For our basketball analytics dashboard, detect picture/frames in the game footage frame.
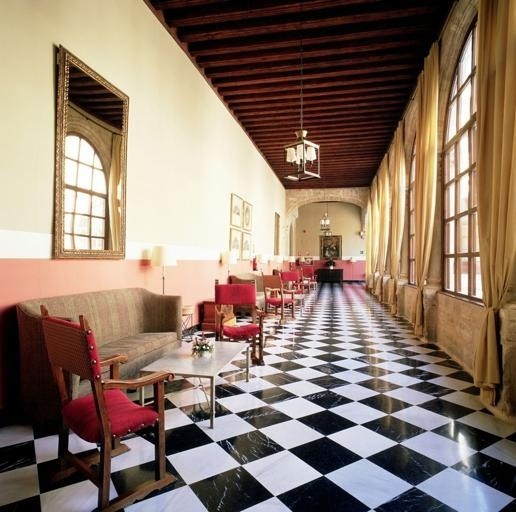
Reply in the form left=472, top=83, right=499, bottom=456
left=319, top=234, right=343, bottom=260
left=227, top=192, right=256, bottom=263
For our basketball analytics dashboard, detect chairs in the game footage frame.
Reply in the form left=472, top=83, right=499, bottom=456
left=38, top=303, right=178, bottom=512
left=213, top=279, right=265, bottom=367
left=262, top=262, right=319, bottom=326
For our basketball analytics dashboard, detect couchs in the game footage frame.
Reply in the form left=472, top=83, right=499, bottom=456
left=15, top=286, right=185, bottom=437
left=229, top=271, right=271, bottom=317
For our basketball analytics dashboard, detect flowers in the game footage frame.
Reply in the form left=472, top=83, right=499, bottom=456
left=190, top=332, right=214, bottom=362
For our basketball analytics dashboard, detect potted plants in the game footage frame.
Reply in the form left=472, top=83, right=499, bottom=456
left=325, top=259, right=336, bottom=271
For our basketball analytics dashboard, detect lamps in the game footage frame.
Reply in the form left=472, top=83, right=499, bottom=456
left=358, top=229, right=366, bottom=240
left=318, top=201, right=333, bottom=240
left=142, top=242, right=179, bottom=297
left=282, top=1, right=324, bottom=185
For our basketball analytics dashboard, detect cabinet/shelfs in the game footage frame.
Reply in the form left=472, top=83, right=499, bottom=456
left=319, top=268, right=344, bottom=289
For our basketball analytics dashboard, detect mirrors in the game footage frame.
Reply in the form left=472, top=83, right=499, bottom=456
left=53, top=44, right=129, bottom=260
